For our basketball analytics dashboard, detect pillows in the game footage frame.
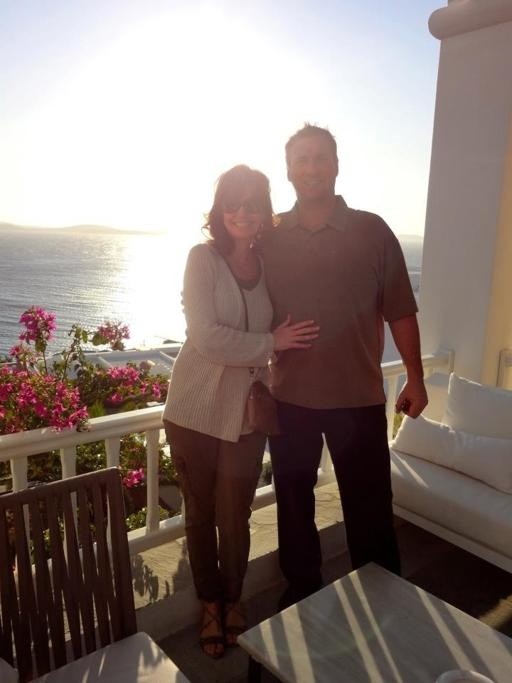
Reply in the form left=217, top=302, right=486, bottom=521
left=388, top=373, right=511, bottom=497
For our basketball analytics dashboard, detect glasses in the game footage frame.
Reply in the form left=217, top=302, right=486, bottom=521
left=216, top=198, right=270, bottom=214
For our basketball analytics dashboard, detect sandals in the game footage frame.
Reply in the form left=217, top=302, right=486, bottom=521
left=225, top=602, right=245, bottom=648
left=200, top=601, right=225, bottom=657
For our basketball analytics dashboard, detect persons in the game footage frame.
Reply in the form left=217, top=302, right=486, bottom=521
left=161, top=164, right=321, bottom=661
left=180, top=121, right=427, bottom=613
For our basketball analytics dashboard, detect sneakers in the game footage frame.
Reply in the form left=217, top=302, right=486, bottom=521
left=277, top=578, right=315, bottom=613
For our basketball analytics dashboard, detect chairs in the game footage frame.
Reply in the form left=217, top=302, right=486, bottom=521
left=387, top=371, right=511, bottom=575
left=0, top=465, right=193, bottom=682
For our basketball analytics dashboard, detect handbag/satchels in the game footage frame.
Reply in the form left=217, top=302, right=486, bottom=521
left=246, top=380, right=281, bottom=437
